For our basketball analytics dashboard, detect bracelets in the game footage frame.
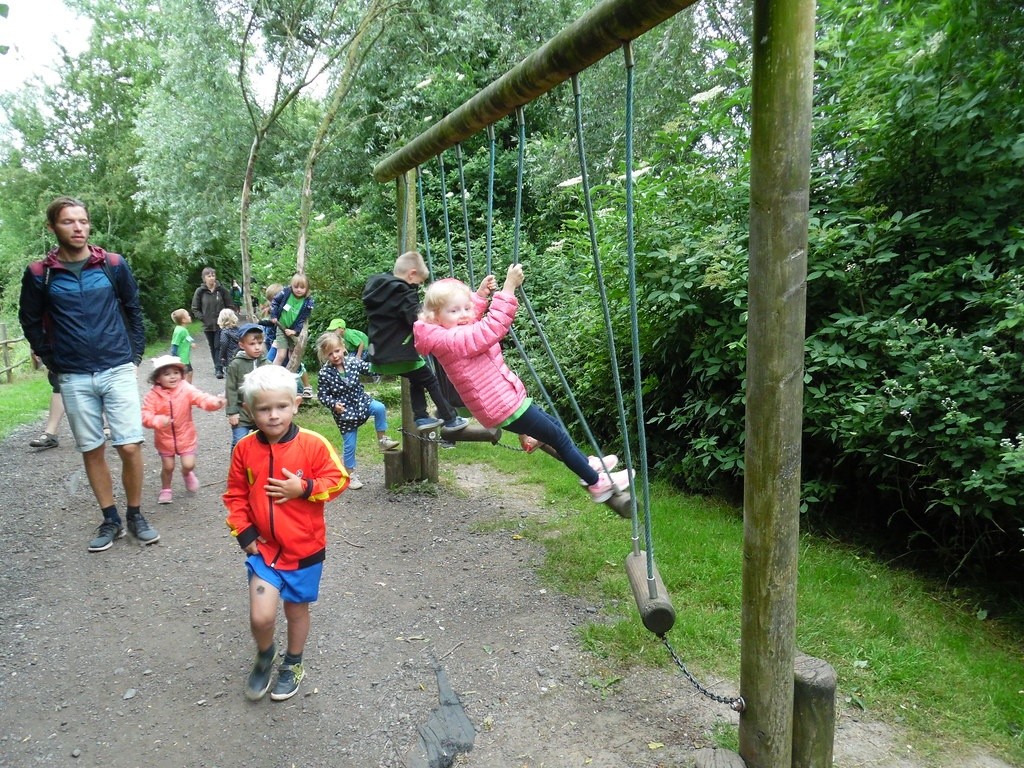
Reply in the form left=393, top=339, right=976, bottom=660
left=301, top=478, right=314, bottom=500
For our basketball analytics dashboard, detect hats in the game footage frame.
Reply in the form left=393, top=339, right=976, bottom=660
left=326, top=318, right=346, bottom=331
left=239, top=323, right=263, bottom=340
left=146, top=355, right=185, bottom=384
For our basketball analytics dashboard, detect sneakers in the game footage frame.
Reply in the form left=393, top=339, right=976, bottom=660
left=378, top=435, right=400, bottom=451
left=416, top=415, right=444, bottom=431
left=442, top=415, right=469, bottom=432
left=184, top=470, right=199, bottom=491
left=88, top=518, right=126, bottom=551
left=271, top=663, right=305, bottom=700
left=348, top=472, right=363, bottom=490
left=159, top=488, right=174, bottom=504
left=125, top=512, right=160, bottom=544
left=579, top=454, right=618, bottom=486
left=588, top=468, right=635, bottom=503
left=245, top=652, right=278, bottom=702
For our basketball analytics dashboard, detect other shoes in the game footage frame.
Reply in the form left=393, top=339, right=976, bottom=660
left=216, top=370, right=224, bottom=378
left=522, top=437, right=545, bottom=453
left=373, top=375, right=380, bottom=383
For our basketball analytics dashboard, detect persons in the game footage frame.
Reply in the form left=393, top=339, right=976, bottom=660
left=17, top=195, right=161, bottom=552
left=230, top=278, right=242, bottom=316
left=142, top=355, right=228, bottom=505
left=29, top=371, right=65, bottom=450
left=269, top=272, right=314, bottom=365
left=258, top=282, right=313, bottom=399
left=250, top=277, right=260, bottom=313
left=192, top=267, right=234, bottom=379
left=413, top=263, right=636, bottom=503
left=363, top=250, right=470, bottom=435
left=326, top=318, right=381, bottom=384
left=224, top=365, right=352, bottom=702
left=217, top=309, right=273, bottom=461
left=170, top=308, right=196, bottom=384
left=316, top=331, right=401, bottom=490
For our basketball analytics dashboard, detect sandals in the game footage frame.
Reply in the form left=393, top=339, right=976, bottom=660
left=302, top=385, right=313, bottom=396
left=31, top=432, right=59, bottom=447
left=103, top=425, right=112, bottom=441
